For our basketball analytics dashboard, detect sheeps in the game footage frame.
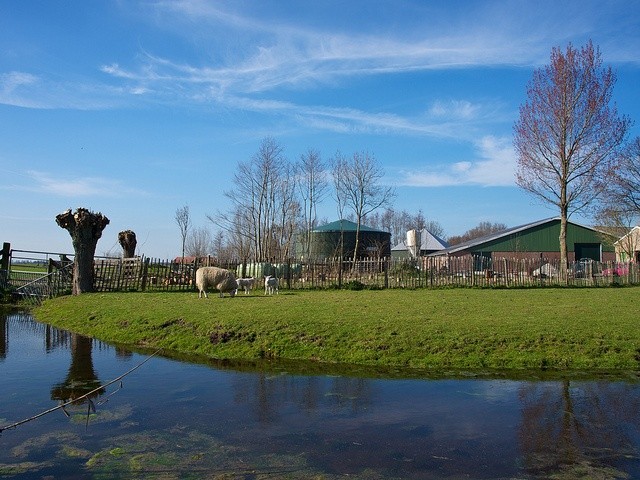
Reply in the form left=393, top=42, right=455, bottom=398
left=263, top=275, right=279, bottom=295
left=196, top=267, right=238, bottom=299
left=235, top=275, right=256, bottom=295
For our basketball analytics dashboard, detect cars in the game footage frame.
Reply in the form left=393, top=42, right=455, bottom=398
left=602, top=263, right=630, bottom=277
